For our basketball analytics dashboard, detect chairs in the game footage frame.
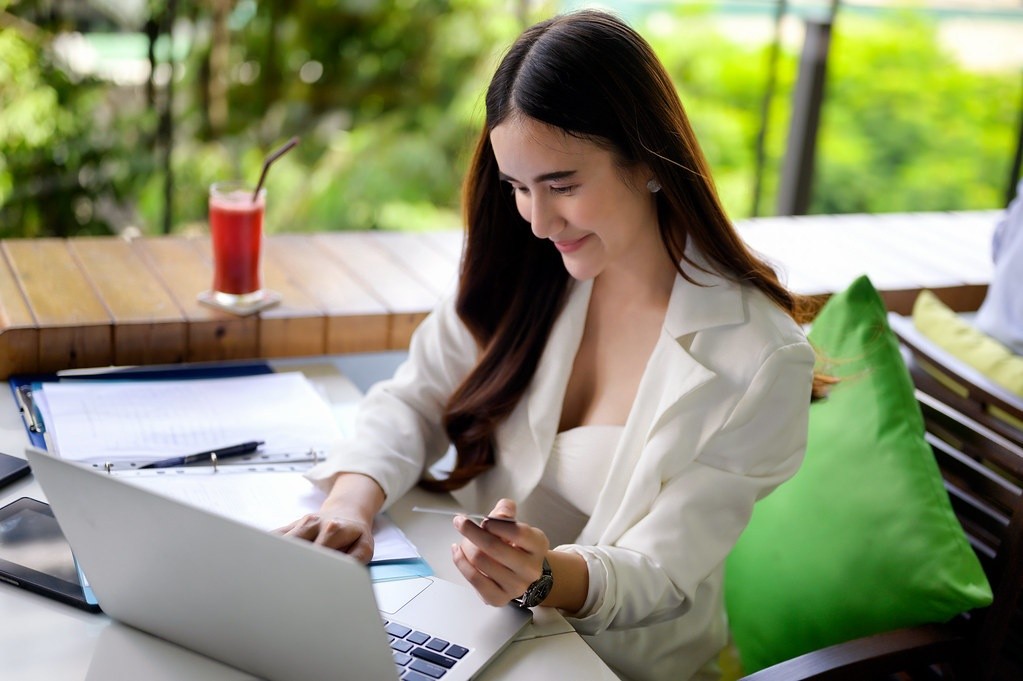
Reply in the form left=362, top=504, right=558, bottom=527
left=709, top=311, right=1023, bottom=681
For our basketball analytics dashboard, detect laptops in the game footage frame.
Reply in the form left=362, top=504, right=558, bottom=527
left=24, top=444, right=534, bottom=680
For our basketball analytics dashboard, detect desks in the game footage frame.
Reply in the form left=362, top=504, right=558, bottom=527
left=0, top=362, right=619, bottom=681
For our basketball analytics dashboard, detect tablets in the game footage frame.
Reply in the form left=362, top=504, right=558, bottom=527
left=0, top=497, right=109, bottom=615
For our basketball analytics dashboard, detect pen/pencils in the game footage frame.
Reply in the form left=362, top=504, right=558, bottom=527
left=137, top=437, right=266, bottom=470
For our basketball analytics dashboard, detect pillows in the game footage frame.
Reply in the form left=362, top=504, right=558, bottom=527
left=913, top=287, right=1023, bottom=478
left=698, top=276, right=993, bottom=672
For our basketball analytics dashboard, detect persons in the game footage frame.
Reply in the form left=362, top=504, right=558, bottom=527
left=268, top=9, right=835, bottom=681
left=975, top=178, right=1023, bottom=355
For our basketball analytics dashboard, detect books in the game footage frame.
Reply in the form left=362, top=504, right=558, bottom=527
left=37, top=371, right=432, bottom=606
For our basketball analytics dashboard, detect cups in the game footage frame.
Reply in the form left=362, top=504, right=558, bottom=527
left=207, top=181, right=266, bottom=305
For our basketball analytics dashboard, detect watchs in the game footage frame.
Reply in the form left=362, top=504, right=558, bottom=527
left=513, top=556, right=554, bottom=608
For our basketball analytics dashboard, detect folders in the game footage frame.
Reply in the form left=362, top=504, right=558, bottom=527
left=7, top=361, right=433, bottom=605
